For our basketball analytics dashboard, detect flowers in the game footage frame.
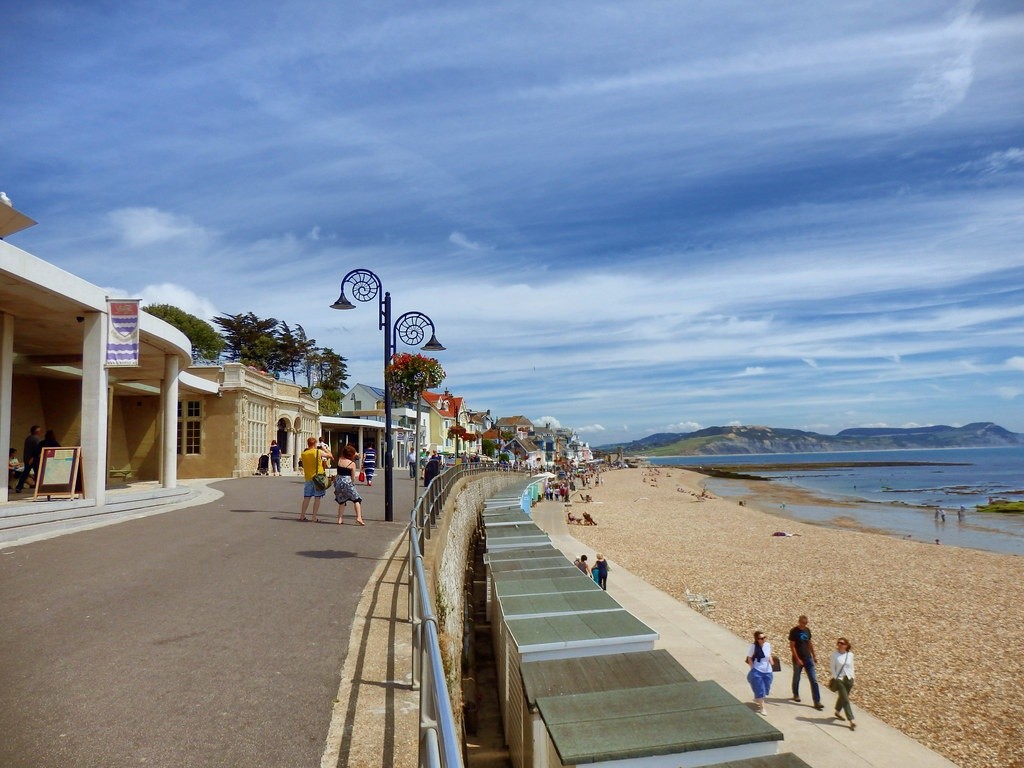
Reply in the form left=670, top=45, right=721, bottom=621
left=384, top=351, right=447, bottom=408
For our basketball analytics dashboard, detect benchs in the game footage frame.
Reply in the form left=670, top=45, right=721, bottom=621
left=109, top=468, right=138, bottom=483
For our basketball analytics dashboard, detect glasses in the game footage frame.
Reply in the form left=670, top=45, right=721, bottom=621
left=758, top=636, right=766, bottom=640
left=837, top=641, right=847, bottom=645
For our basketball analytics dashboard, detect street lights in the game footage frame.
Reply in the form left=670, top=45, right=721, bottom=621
left=438, top=397, right=475, bottom=458
left=329, top=266, right=448, bottom=522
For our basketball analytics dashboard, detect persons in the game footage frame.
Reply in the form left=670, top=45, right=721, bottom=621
left=545, top=463, right=602, bottom=501
left=299, top=437, right=332, bottom=523
left=362, top=443, right=377, bottom=486
left=787, top=615, right=824, bottom=708
left=745, top=631, right=774, bottom=716
left=591, top=553, right=608, bottom=590
left=8, top=424, right=62, bottom=493
left=461, top=450, right=480, bottom=469
left=830, top=637, right=856, bottom=730
left=419, top=448, right=442, bottom=480
left=773, top=531, right=802, bottom=536
left=934, top=505, right=965, bottom=522
left=316, top=437, right=328, bottom=461
left=495, top=459, right=508, bottom=468
left=333, top=446, right=366, bottom=526
left=406, top=447, right=416, bottom=480
left=267, top=439, right=282, bottom=476
left=576, top=555, right=591, bottom=575
left=568, top=512, right=597, bottom=525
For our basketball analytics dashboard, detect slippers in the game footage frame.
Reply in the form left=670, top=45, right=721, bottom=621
left=299, top=517, right=310, bottom=522
left=311, top=518, right=321, bottom=522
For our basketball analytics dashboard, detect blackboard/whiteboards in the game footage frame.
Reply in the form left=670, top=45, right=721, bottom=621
left=34, top=447, right=85, bottom=496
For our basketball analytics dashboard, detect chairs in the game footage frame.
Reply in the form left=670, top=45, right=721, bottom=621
left=584, top=515, right=593, bottom=525
left=580, top=493, right=592, bottom=503
left=566, top=513, right=583, bottom=525
left=683, top=586, right=718, bottom=616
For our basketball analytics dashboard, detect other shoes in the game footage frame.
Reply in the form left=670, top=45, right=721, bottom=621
left=794, top=694, right=801, bottom=701
left=814, top=702, right=824, bottom=708
left=367, top=482, right=370, bottom=486
left=355, top=518, right=365, bottom=526
left=761, top=708, right=767, bottom=716
left=851, top=723, right=856, bottom=730
left=338, top=520, right=343, bottom=524
left=835, top=712, right=845, bottom=720
left=755, top=705, right=760, bottom=712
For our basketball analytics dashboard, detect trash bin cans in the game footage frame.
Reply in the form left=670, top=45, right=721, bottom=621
left=424, top=461, right=441, bottom=487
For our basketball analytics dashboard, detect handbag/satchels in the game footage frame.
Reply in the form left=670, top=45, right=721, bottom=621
left=312, top=474, right=332, bottom=491
left=829, top=678, right=838, bottom=692
left=359, top=468, right=365, bottom=481
left=606, top=564, right=611, bottom=572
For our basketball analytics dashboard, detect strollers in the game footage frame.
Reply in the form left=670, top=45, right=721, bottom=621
left=254, top=454, right=269, bottom=476
